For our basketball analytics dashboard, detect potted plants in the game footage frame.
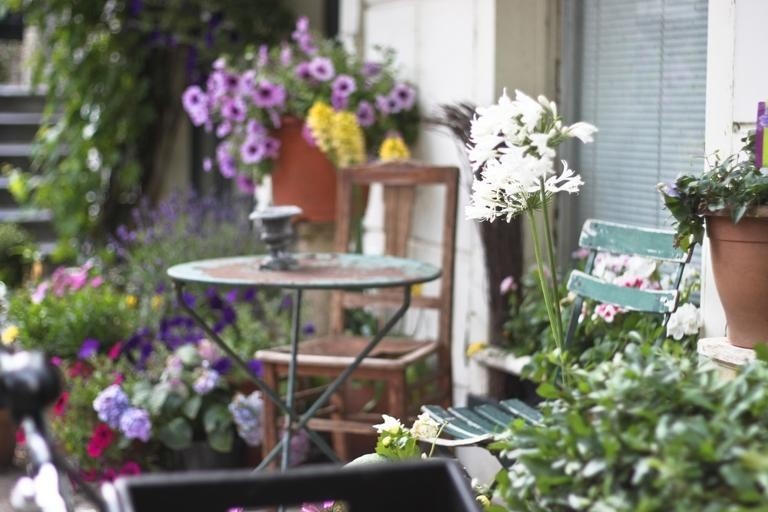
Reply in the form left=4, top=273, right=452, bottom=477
left=656, top=146, right=768, bottom=347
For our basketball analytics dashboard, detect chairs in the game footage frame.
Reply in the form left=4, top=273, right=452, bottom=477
left=420, top=219, right=696, bottom=450
